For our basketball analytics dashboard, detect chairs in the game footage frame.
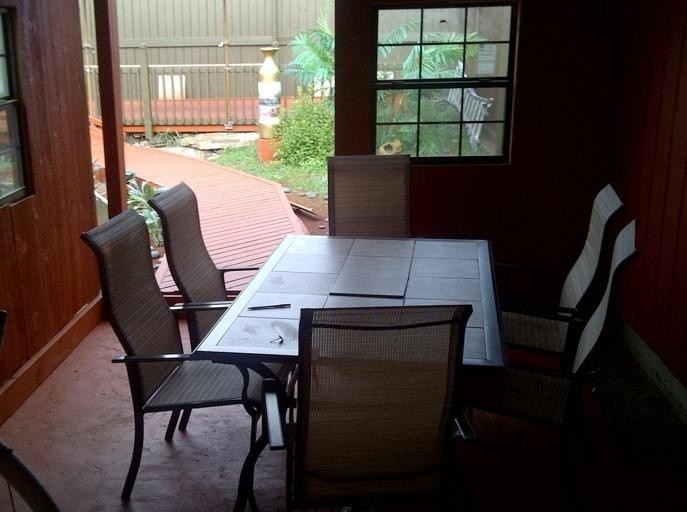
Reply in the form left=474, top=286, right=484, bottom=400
left=260, top=306, right=481, bottom=512
left=498, top=180, right=624, bottom=372
left=470, top=217, right=641, bottom=480
left=79, top=210, right=290, bottom=511
left=151, top=182, right=290, bottom=430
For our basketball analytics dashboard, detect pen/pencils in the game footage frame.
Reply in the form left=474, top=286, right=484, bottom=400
left=248, top=304, right=291, bottom=309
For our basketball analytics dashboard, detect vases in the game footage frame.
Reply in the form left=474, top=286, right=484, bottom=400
left=257, top=47, right=281, bottom=138
